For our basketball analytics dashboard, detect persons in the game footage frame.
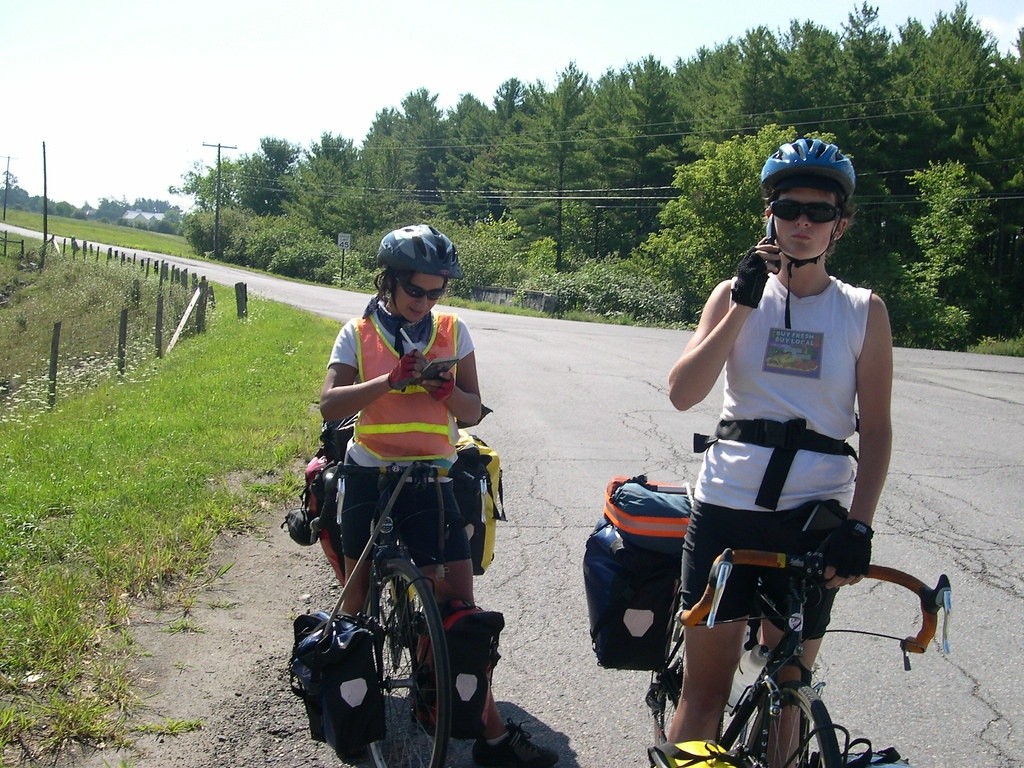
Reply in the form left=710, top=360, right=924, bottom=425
left=668, top=139, right=893, bottom=768
left=319, top=223, right=560, bottom=768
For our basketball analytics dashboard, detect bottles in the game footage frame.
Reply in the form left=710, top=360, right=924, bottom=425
left=724, top=644, right=770, bottom=715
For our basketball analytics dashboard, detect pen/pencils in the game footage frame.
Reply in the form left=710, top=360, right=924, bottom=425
left=400, top=327, right=430, bottom=364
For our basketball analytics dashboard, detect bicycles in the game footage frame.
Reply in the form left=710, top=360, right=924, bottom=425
left=645, top=548, right=954, bottom=767
left=322, top=460, right=487, bottom=767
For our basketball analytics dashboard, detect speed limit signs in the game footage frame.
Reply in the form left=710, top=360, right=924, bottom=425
left=338, top=234, right=351, bottom=249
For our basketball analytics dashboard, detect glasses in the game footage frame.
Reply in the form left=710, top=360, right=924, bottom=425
left=770, top=200, right=843, bottom=224
left=395, top=275, right=445, bottom=302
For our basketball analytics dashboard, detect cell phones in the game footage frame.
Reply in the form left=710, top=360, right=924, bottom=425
left=766, top=213, right=781, bottom=275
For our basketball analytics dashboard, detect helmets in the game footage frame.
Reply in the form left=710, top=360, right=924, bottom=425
left=378, top=224, right=463, bottom=280
left=760, top=139, right=856, bottom=205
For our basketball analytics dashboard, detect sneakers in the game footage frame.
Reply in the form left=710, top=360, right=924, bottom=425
left=473, top=723, right=559, bottom=768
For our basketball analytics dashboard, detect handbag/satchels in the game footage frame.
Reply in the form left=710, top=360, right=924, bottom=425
left=288, top=608, right=388, bottom=755
left=414, top=600, right=506, bottom=737
left=584, top=477, right=691, bottom=671
left=306, top=442, right=346, bottom=588
left=448, top=429, right=508, bottom=575
